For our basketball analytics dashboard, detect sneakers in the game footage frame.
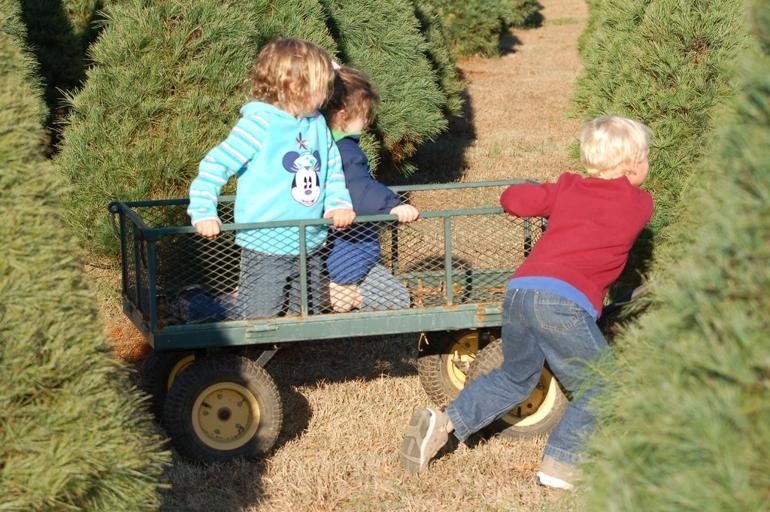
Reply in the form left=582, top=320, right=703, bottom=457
left=400, top=407, right=450, bottom=473
left=169, top=283, right=208, bottom=317
left=536, top=456, right=574, bottom=492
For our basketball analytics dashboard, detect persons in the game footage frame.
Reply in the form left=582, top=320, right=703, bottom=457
left=398, top=113, right=655, bottom=489
left=317, top=63, right=420, bottom=312
left=173, top=36, right=356, bottom=319
